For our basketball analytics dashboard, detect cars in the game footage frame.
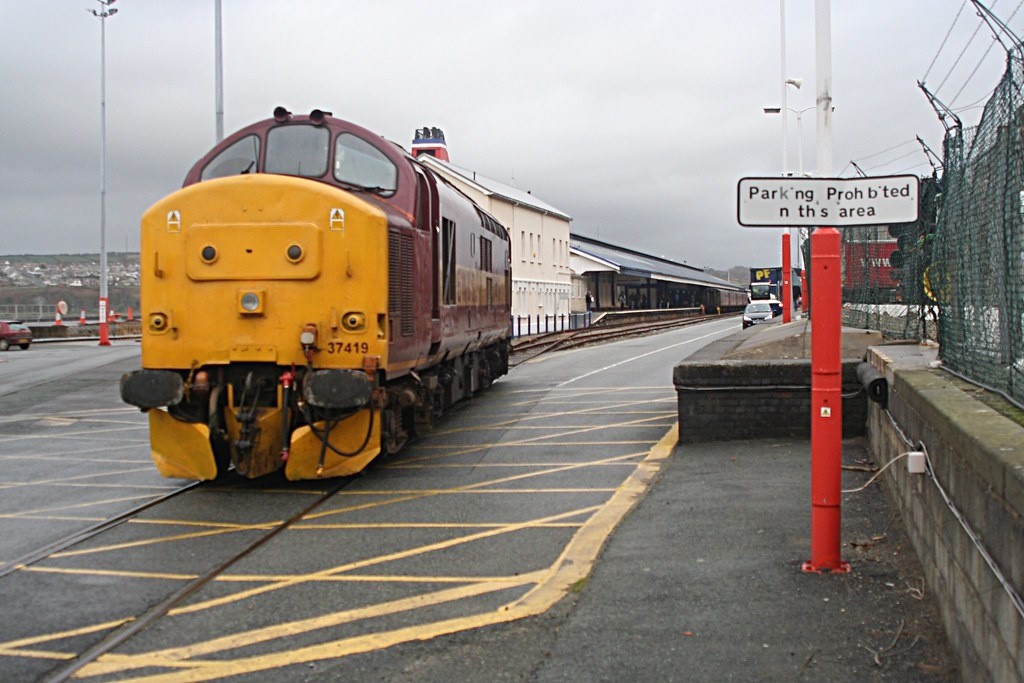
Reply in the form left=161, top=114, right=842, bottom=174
left=0, top=320, right=33, bottom=351
left=742, top=303, right=774, bottom=331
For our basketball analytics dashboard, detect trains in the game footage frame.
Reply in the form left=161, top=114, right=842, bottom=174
left=122, top=109, right=513, bottom=482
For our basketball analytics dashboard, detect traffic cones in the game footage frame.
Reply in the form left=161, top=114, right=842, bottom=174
left=126, top=306, right=136, bottom=322
left=52, top=307, right=67, bottom=327
left=77, top=307, right=89, bottom=325
left=108, top=307, right=117, bottom=323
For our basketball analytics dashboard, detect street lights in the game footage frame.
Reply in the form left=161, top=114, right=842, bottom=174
left=759, top=100, right=852, bottom=321
left=88, top=0, right=118, bottom=348
left=778, top=76, right=802, bottom=329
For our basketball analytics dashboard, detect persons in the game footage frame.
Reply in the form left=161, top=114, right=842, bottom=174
left=619, top=291, right=647, bottom=310
left=658, top=290, right=699, bottom=309
left=586, top=291, right=592, bottom=311
